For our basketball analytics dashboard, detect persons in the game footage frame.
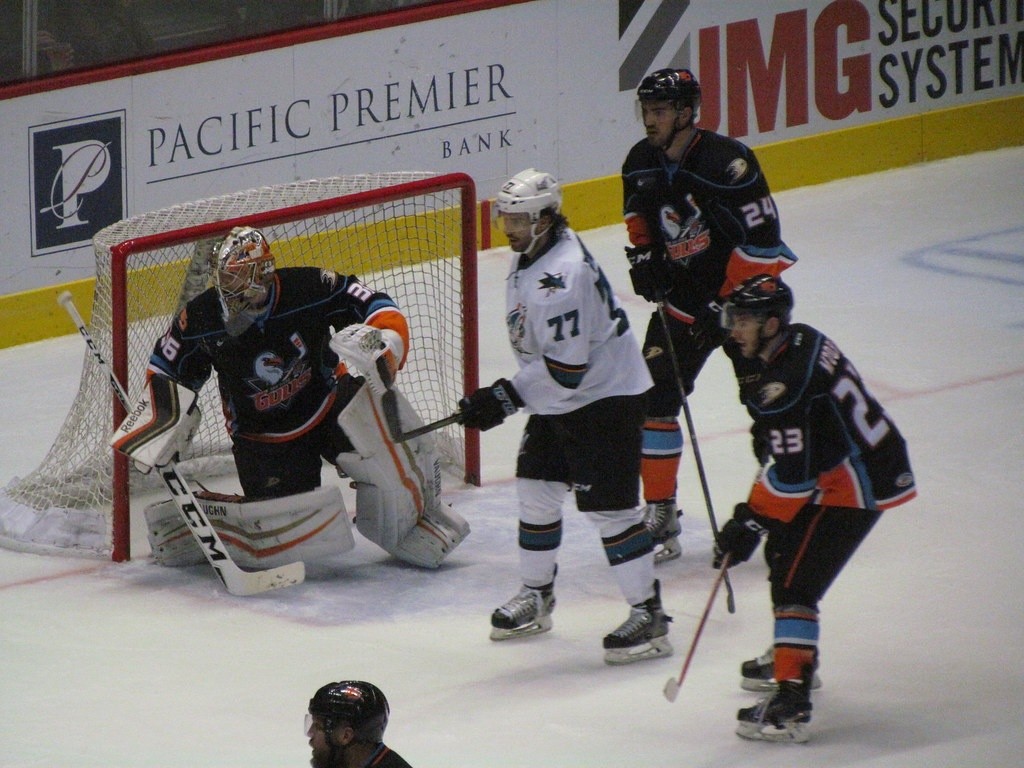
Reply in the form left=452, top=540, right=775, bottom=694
left=458, top=166, right=676, bottom=665
left=620, top=66, right=799, bottom=565
left=710, top=274, right=919, bottom=744
left=305, top=680, right=414, bottom=768
left=109, top=226, right=471, bottom=570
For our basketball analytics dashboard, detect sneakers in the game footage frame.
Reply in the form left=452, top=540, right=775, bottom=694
left=634, top=496, right=683, bottom=564
left=604, top=579, right=673, bottom=663
left=740, top=647, right=821, bottom=690
left=734, top=680, right=813, bottom=742
left=490, top=564, right=559, bottom=640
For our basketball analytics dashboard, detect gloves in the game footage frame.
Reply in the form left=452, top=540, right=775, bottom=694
left=689, top=294, right=730, bottom=352
left=713, top=502, right=770, bottom=568
left=624, top=240, right=670, bottom=304
left=458, top=377, right=525, bottom=431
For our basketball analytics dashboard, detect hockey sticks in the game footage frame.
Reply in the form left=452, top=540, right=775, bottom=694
left=57, top=287, right=306, bottom=597
left=661, top=548, right=733, bottom=704
left=380, top=390, right=460, bottom=444
left=657, top=294, right=736, bottom=614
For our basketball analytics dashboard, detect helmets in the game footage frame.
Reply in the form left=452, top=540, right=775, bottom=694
left=635, top=68, right=701, bottom=106
left=309, top=680, right=391, bottom=748
left=212, top=226, right=276, bottom=337
left=726, top=273, right=793, bottom=315
left=495, top=168, right=563, bottom=212
